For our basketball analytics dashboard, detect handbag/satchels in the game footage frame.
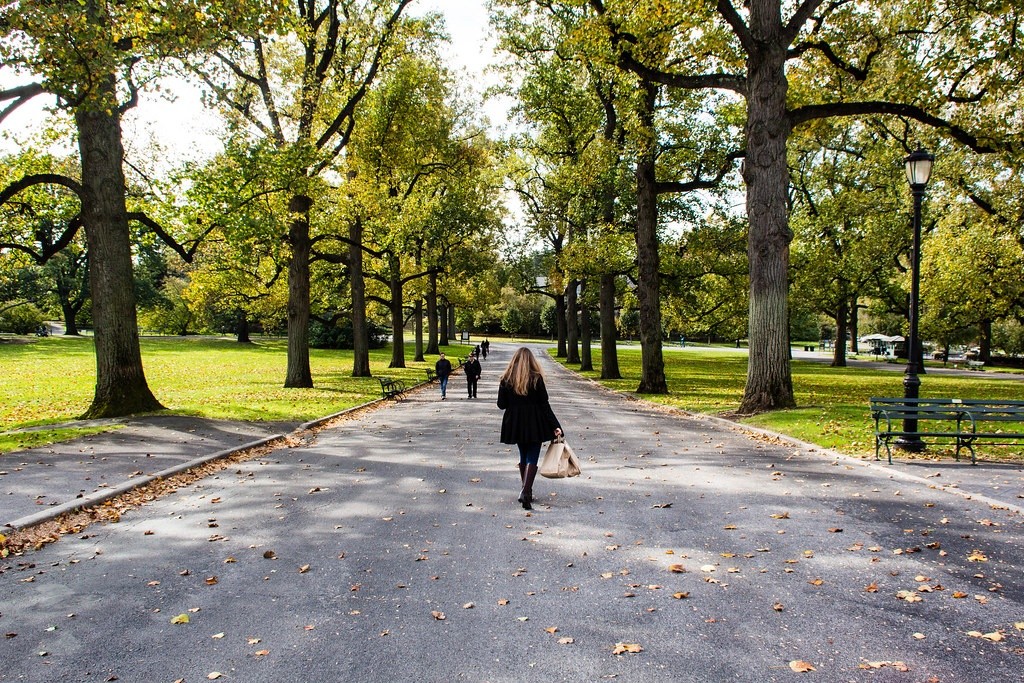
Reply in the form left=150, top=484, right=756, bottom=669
left=542, top=439, right=568, bottom=477
left=558, top=435, right=582, bottom=480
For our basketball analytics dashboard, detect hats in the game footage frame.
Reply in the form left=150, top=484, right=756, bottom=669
left=468, top=353, right=474, bottom=358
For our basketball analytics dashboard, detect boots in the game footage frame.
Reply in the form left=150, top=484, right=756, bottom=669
left=517, top=461, right=538, bottom=510
left=518, top=461, right=534, bottom=502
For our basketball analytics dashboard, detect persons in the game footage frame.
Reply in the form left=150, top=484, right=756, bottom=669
left=260, top=327, right=284, bottom=339
left=35, top=323, right=47, bottom=338
left=221, top=324, right=226, bottom=336
left=497, top=348, right=564, bottom=510
left=464, top=351, right=482, bottom=399
left=436, top=352, right=452, bottom=401
left=474, top=339, right=490, bottom=361
left=735, top=337, right=741, bottom=348
left=880, top=344, right=886, bottom=356
left=232, top=326, right=238, bottom=338
left=680, top=333, right=686, bottom=348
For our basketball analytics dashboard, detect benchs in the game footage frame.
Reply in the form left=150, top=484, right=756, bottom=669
left=425, top=368, right=439, bottom=384
left=379, top=377, right=406, bottom=404
left=869, top=398, right=1024, bottom=466
